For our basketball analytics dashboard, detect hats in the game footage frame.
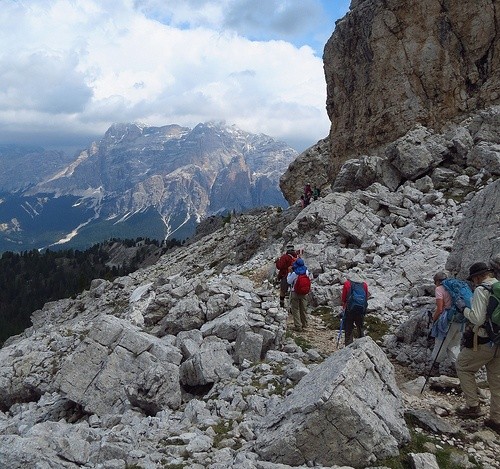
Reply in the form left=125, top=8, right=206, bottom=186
left=287, top=245, right=294, bottom=250
left=346, top=267, right=367, bottom=284
left=293, top=259, right=307, bottom=275
left=466, top=262, right=491, bottom=281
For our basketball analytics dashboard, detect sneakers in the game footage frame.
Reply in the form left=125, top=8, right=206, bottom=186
left=484, top=418, right=500, bottom=434
left=457, top=404, right=485, bottom=420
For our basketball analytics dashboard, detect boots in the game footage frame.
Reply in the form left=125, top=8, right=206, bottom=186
left=426, top=362, right=440, bottom=377
left=445, top=362, right=458, bottom=378
left=280, top=296, right=284, bottom=307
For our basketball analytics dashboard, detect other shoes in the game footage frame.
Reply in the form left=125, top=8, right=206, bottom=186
left=293, top=325, right=310, bottom=332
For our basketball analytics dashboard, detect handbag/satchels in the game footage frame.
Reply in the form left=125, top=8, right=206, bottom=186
left=463, top=330, right=491, bottom=348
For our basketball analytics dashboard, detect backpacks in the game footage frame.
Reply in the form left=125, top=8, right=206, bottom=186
left=288, top=254, right=299, bottom=266
left=476, top=281, right=500, bottom=346
left=345, top=282, right=368, bottom=321
left=440, top=278, right=474, bottom=323
left=294, top=274, right=310, bottom=295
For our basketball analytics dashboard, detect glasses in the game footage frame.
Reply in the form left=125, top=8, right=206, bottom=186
left=434, top=282, right=436, bottom=284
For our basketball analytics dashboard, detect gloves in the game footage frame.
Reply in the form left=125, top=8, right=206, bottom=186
left=455, top=296, right=467, bottom=314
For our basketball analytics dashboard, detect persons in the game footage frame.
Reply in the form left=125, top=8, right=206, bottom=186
left=276, top=245, right=296, bottom=308
left=429, top=272, right=461, bottom=378
left=341, top=268, right=368, bottom=349
left=287, top=259, right=310, bottom=331
left=454, top=261, right=500, bottom=435
